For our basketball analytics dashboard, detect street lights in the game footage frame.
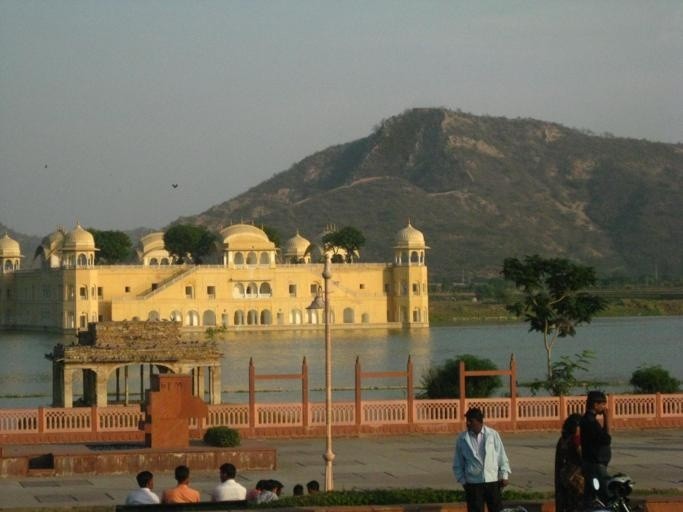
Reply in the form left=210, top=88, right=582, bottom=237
left=313, top=225, right=340, bottom=455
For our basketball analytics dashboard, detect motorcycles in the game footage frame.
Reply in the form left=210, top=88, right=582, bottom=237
left=499, top=471, right=648, bottom=512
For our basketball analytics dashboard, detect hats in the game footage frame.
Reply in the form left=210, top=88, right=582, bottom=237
left=464, top=407, right=486, bottom=418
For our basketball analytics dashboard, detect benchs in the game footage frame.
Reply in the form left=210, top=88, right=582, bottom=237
left=116, top=499, right=249, bottom=511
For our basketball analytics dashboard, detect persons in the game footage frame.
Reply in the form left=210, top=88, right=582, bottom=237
left=582, top=390, right=614, bottom=493
left=256, top=478, right=284, bottom=505
left=271, top=480, right=284, bottom=496
left=125, top=470, right=160, bottom=505
left=553, top=411, right=601, bottom=510
left=305, top=479, right=320, bottom=495
left=292, top=484, right=304, bottom=497
left=161, top=465, right=200, bottom=505
left=211, top=463, right=247, bottom=501
left=452, top=407, right=513, bottom=512
left=245, top=479, right=267, bottom=500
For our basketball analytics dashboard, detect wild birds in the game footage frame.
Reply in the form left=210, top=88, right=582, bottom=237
left=171, top=183, right=178, bottom=189
